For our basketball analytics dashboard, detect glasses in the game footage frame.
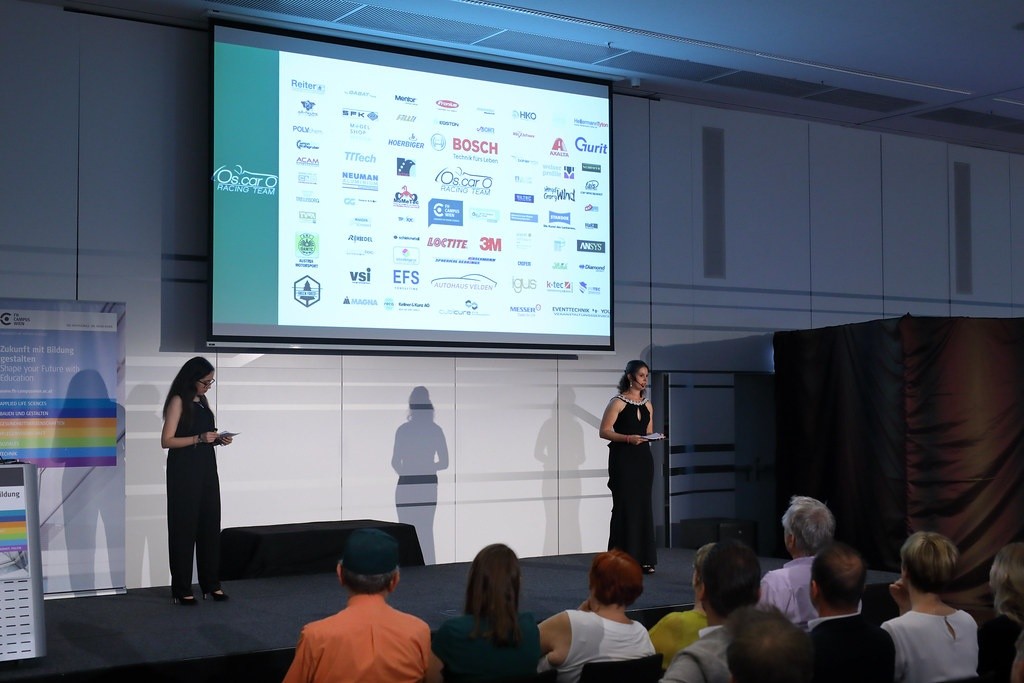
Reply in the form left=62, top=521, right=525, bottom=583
left=197, top=378, right=215, bottom=389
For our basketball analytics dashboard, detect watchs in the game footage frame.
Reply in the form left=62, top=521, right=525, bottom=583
left=198, top=434, right=203, bottom=443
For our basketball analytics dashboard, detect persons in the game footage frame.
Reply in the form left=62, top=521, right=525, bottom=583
left=283, top=529, right=445, bottom=683
left=647, top=543, right=717, bottom=671
left=600, top=359, right=664, bottom=574
left=725, top=611, right=813, bottom=683
left=976, top=544, right=1024, bottom=683
left=880, top=531, right=979, bottom=683
left=537, top=550, right=656, bottom=683
left=757, top=496, right=862, bottom=631
left=432, top=544, right=541, bottom=683
left=161, top=357, right=232, bottom=605
left=659, top=540, right=762, bottom=683
left=801, top=542, right=896, bottom=683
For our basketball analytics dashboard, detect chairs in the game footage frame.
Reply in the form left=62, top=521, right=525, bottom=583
left=581, top=654, right=665, bottom=683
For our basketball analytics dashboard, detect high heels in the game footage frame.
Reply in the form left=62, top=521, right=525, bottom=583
left=174, top=596, right=199, bottom=607
left=203, top=590, right=230, bottom=601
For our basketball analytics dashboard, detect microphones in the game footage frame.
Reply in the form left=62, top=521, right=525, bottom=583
left=634, top=380, right=644, bottom=388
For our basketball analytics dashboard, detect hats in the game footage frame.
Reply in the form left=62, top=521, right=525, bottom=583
left=348, top=527, right=399, bottom=574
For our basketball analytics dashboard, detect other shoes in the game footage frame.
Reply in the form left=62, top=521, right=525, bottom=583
left=642, top=563, right=655, bottom=575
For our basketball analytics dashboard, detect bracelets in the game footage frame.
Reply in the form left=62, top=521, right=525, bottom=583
left=193, top=436, right=196, bottom=447
left=626, top=435, right=630, bottom=443
left=216, top=439, right=221, bottom=445
